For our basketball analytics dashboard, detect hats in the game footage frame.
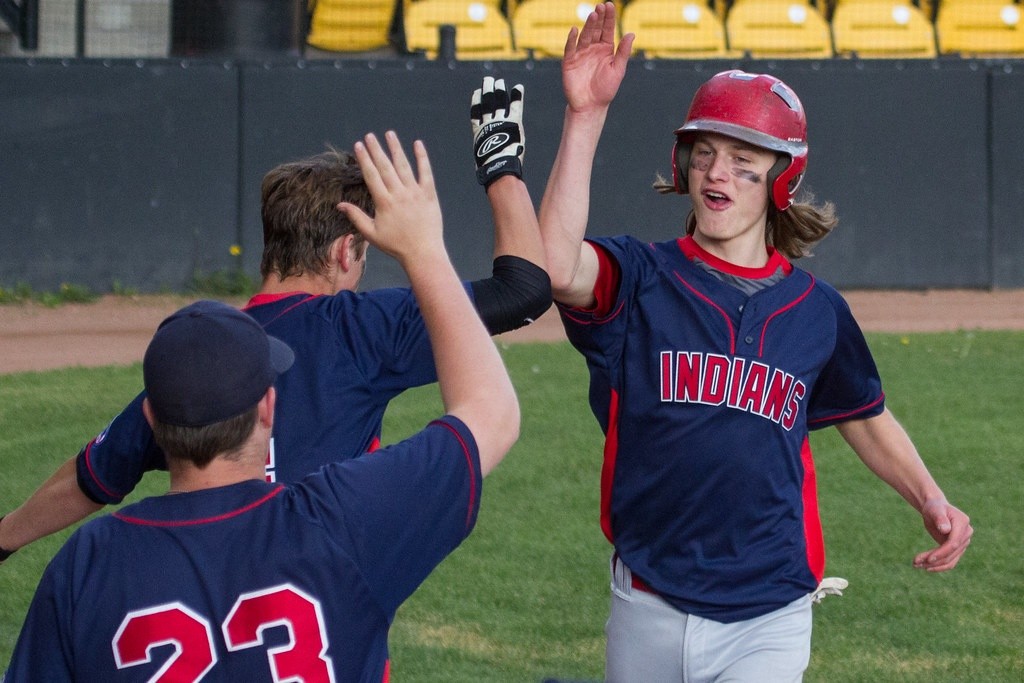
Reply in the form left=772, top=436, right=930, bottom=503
left=143, top=300, right=294, bottom=428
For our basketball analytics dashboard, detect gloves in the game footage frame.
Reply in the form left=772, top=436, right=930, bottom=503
left=472, top=76, right=525, bottom=191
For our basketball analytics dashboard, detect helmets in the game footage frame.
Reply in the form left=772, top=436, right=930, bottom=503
left=671, top=69, right=808, bottom=209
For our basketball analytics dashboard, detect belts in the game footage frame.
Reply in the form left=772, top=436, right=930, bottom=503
left=610, top=554, right=658, bottom=595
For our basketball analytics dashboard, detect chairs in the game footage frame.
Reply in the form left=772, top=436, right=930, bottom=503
left=305, top=0, right=1024, bottom=58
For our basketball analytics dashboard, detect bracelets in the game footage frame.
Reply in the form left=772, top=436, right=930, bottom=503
left=0, top=514, right=18, bottom=562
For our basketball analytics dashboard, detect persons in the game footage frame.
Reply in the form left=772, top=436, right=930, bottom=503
left=538, top=2, right=974, bottom=683
left=1, top=129, right=522, bottom=683
left=1, top=74, right=554, bottom=564
